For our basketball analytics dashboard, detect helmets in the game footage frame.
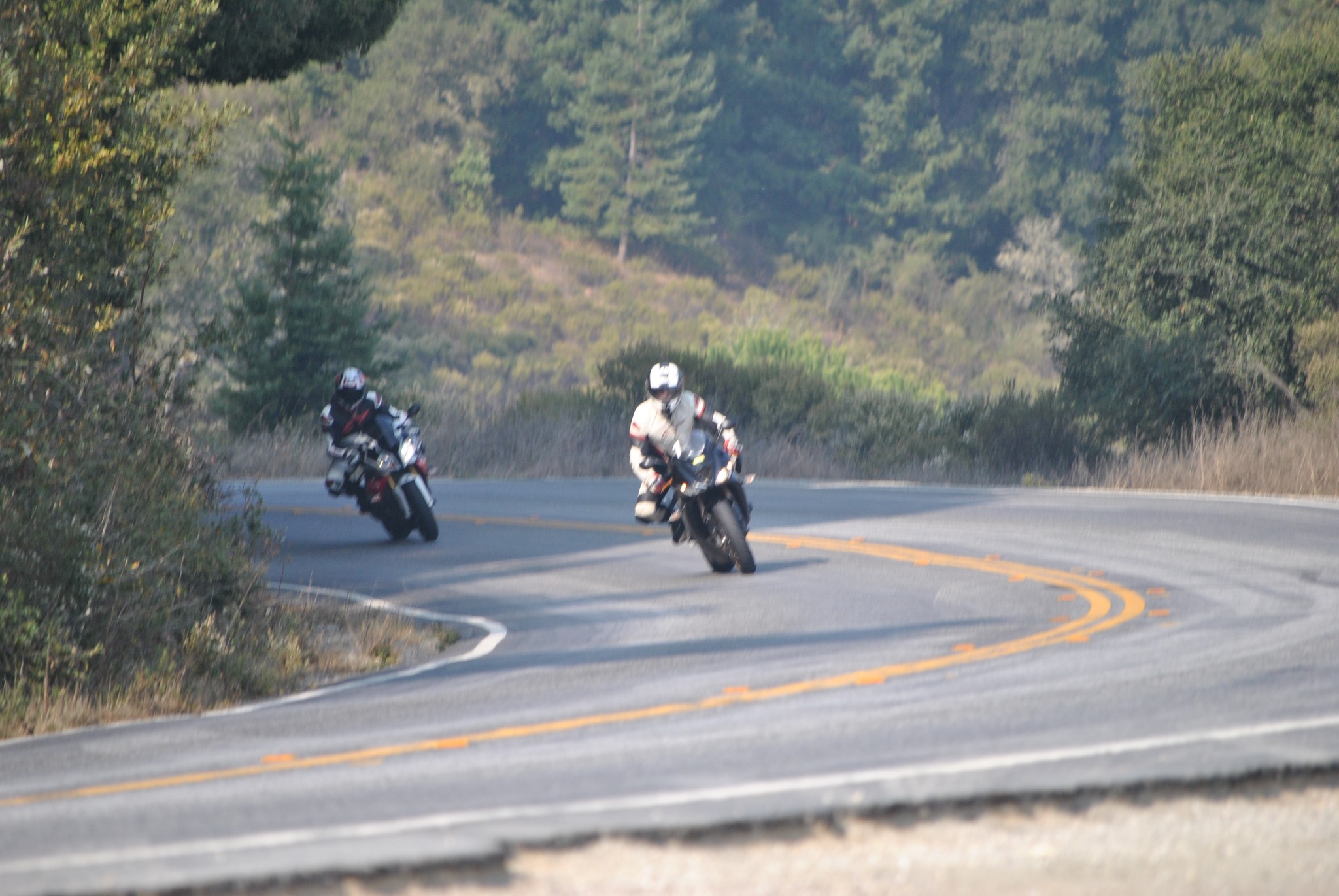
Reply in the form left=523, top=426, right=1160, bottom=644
left=646, top=361, right=683, bottom=414
left=335, top=366, right=366, bottom=411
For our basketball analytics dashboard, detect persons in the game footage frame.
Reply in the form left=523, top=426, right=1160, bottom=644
left=318, top=367, right=430, bottom=513
left=628, top=361, right=743, bottom=544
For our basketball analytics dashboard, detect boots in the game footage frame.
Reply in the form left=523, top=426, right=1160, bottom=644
left=660, top=509, right=687, bottom=543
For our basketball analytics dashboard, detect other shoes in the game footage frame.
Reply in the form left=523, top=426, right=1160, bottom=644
left=357, top=489, right=370, bottom=512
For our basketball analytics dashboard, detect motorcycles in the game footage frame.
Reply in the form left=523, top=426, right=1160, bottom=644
left=332, top=401, right=439, bottom=542
left=639, top=416, right=755, bottom=574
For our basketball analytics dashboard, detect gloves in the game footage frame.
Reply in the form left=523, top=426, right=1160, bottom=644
left=646, top=476, right=664, bottom=495
left=725, top=438, right=743, bottom=455
